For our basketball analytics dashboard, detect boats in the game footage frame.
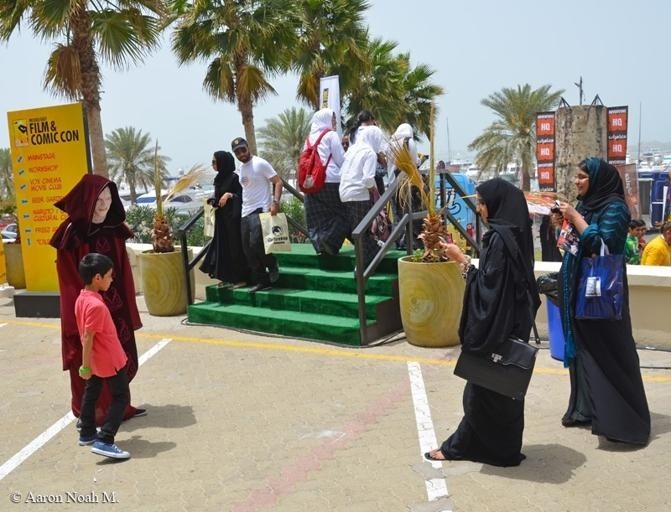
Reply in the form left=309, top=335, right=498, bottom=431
left=447, top=152, right=671, bottom=179
left=116, top=187, right=214, bottom=210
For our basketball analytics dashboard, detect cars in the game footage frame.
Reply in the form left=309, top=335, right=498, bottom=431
left=0, top=223, right=18, bottom=243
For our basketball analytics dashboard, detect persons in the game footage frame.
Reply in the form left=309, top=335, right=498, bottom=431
left=231, top=138, right=283, bottom=293
left=199, top=151, right=247, bottom=288
left=50, top=174, right=147, bottom=421
left=303, top=108, right=346, bottom=256
left=338, top=126, right=384, bottom=273
left=385, top=123, right=427, bottom=250
left=558, top=157, right=650, bottom=444
left=349, top=110, right=387, bottom=195
left=624, top=221, right=671, bottom=266
left=424, top=179, right=541, bottom=468
left=74, top=252, right=130, bottom=459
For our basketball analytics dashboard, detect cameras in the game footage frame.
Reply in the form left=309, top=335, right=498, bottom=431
left=551, top=199, right=562, bottom=215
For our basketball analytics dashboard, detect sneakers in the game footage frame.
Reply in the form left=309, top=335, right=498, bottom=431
left=135, top=408, right=146, bottom=417
left=92, top=442, right=131, bottom=459
left=78, top=433, right=99, bottom=446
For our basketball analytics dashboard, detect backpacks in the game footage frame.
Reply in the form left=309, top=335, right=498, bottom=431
left=297, top=128, right=332, bottom=193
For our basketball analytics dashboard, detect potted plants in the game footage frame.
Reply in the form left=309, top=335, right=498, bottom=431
left=138, top=135, right=207, bottom=317
left=0, top=148, right=25, bottom=288
left=379, top=100, right=469, bottom=349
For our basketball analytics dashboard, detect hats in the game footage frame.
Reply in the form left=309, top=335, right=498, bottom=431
left=232, top=137, right=247, bottom=152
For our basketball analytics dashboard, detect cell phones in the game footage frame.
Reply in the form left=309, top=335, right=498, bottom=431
left=437, top=236, right=447, bottom=243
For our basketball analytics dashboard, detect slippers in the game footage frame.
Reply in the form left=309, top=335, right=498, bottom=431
left=424, top=447, right=445, bottom=460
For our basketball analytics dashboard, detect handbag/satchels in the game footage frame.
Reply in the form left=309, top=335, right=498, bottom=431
left=203, top=199, right=216, bottom=238
left=371, top=214, right=393, bottom=242
left=454, top=329, right=538, bottom=401
left=576, top=240, right=627, bottom=320
left=259, top=212, right=291, bottom=255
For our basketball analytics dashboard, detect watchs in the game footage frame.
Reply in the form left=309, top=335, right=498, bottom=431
left=273, top=200, right=280, bottom=205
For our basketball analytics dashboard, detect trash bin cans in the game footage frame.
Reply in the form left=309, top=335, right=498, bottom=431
left=536, top=272, right=565, bottom=361
